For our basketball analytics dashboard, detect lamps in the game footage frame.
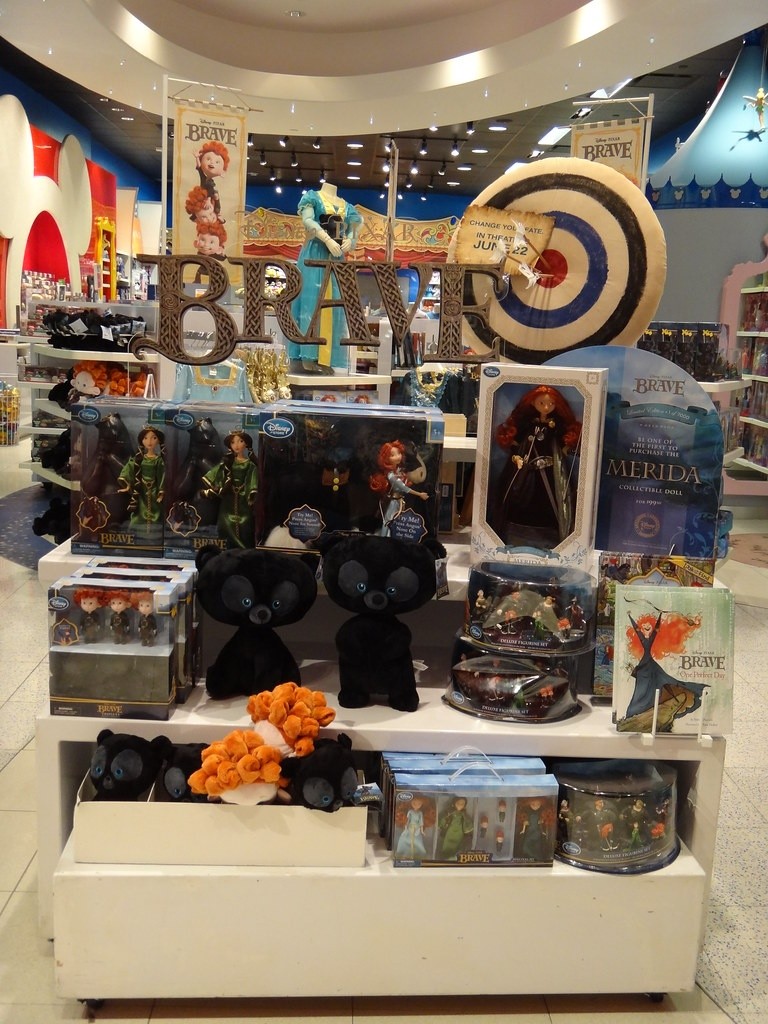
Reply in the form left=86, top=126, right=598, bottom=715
left=244, top=117, right=475, bottom=201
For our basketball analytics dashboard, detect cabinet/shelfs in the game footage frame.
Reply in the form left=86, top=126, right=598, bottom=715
left=288, top=316, right=437, bottom=407
left=698, top=376, right=753, bottom=464
left=34, top=396, right=728, bottom=1019
left=16, top=334, right=159, bottom=492
left=731, top=283, right=768, bottom=473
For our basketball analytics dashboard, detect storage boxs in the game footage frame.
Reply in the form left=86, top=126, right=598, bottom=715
left=468, top=359, right=609, bottom=572
left=42, top=554, right=735, bottom=881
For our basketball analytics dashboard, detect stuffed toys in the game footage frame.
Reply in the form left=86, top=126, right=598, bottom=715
left=32, top=361, right=147, bottom=545
left=318, top=529, right=447, bottom=711
left=89, top=683, right=358, bottom=813
left=194, top=538, right=320, bottom=700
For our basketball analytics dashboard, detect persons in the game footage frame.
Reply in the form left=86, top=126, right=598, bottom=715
left=747, top=88, right=768, bottom=129
left=496, top=386, right=582, bottom=545
left=285, top=181, right=362, bottom=376
left=395, top=792, right=671, bottom=865
left=116, top=427, right=259, bottom=547
left=460, top=589, right=584, bottom=710
left=369, top=440, right=430, bottom=539
left=73, top=587, right=157, bottom=647
